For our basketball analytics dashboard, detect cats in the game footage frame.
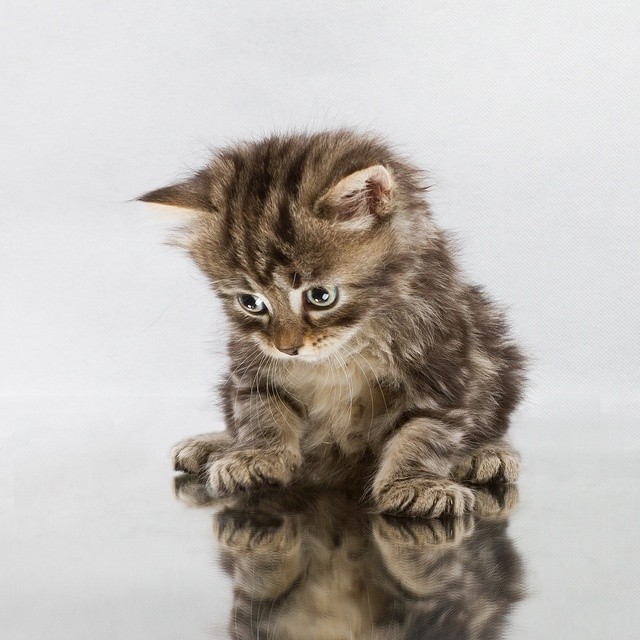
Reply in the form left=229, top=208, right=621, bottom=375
left=123, top=123, right=537, bottom=521
left=172, top=473, right=531, bottom=640
